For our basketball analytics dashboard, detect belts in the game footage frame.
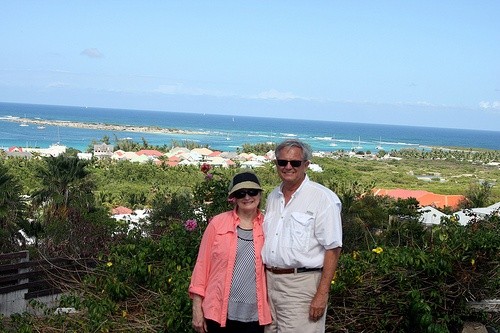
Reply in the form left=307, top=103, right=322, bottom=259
left=264, top=264, right=323, bottom=274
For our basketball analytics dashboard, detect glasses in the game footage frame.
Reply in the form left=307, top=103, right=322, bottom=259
left=234, top=189, right=260, bottom=199
left=277, top=160, right=303, bottom=168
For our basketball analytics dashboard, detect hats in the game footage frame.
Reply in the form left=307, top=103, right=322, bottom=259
left=228, top=169, right=264, bottom=199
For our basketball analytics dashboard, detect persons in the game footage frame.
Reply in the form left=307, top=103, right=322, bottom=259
left=260, top=138, right=343, bottom=333
left=188, top=171, right=274, bottom=333
